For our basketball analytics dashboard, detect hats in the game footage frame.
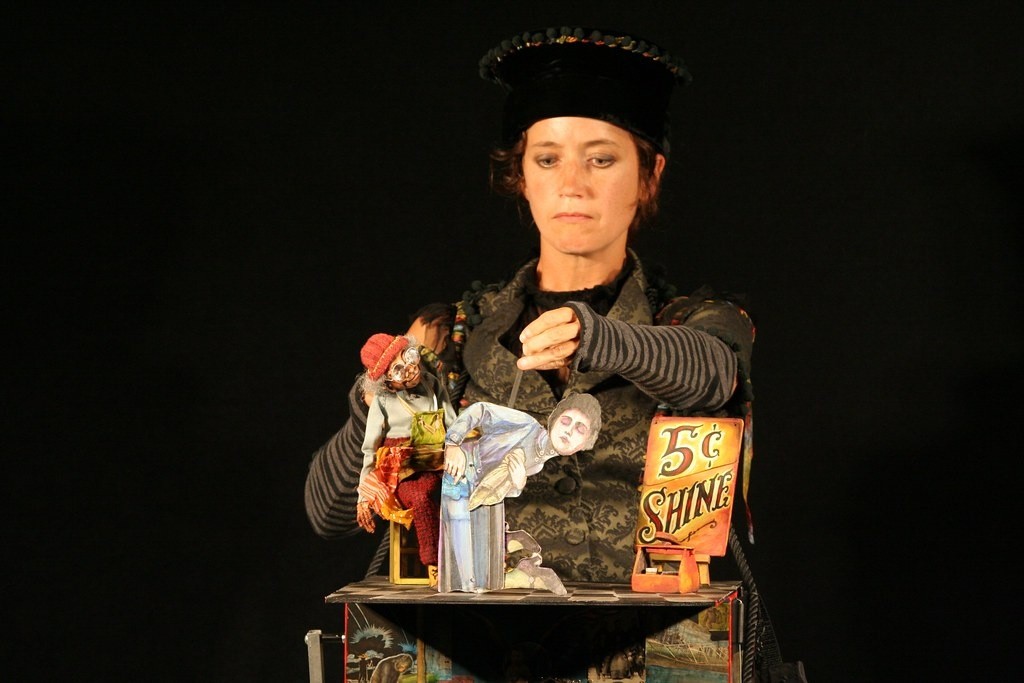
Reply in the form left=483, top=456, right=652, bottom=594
left=480, top=27, right=693, bottom=153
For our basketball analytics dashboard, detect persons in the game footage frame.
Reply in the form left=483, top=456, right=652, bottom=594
left=358, top=334, right=459, bottom=579
left=305, top=28, right=758, bottom=581
